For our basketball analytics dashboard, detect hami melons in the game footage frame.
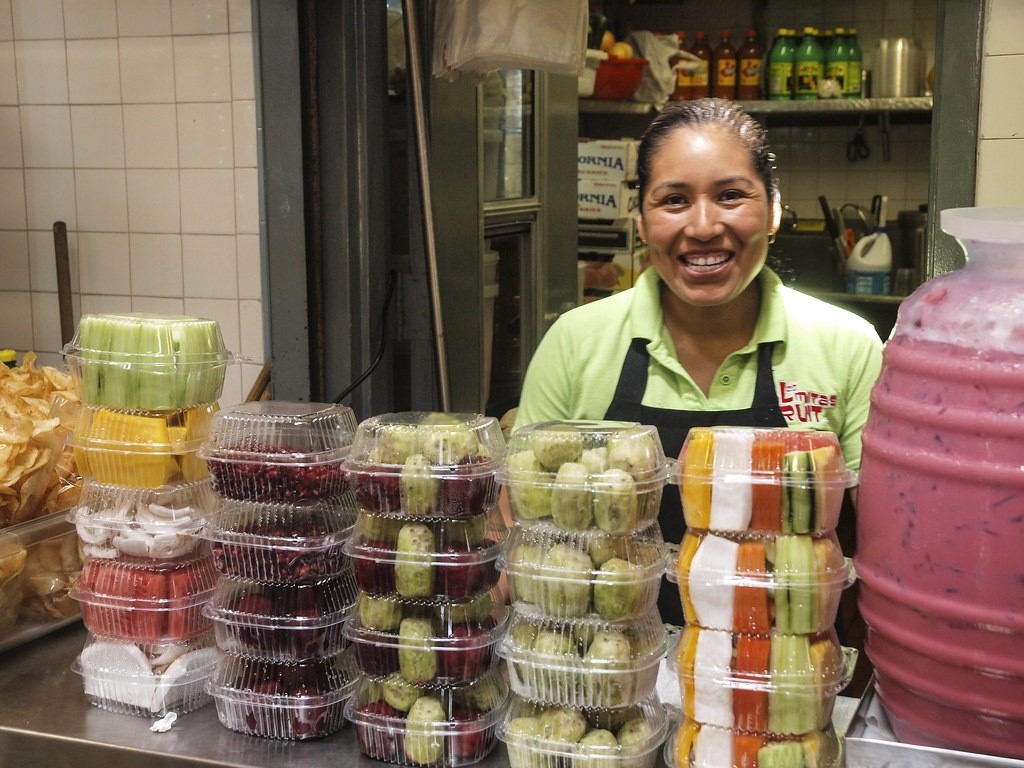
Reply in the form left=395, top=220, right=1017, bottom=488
left=808, top=445, right=848, bottom=768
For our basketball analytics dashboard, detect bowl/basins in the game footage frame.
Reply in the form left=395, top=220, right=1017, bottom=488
left=577, top=48, right=650, bottom=100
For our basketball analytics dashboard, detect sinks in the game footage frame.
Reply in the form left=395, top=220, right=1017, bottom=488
left=764, top=227, right=922, bottom=344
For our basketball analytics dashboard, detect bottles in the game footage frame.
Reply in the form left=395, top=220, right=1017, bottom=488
left=711, top=29, right=737, bottom=99
left=852, top=204, right=1024, bottom=761
left=652, top=31, right=689, bottom=100
left=765, top=27, right=863, bottom=101
left=690, top=32, right=711, bottom=99
left=738, top=29, right=762, bottom=100
left=844, top=226, right=892, bottom=296
left=913, top=204, right=928, bottom=287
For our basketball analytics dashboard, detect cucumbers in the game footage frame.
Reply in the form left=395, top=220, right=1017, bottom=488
left=757, top=450, right=813, bottom=768
left=77, top=316, right=226, bottom=410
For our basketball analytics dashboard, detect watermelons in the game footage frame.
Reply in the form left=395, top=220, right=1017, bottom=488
left=76, top=561, right=222, bottom=639
left=755, top=431, right=840, bottom=454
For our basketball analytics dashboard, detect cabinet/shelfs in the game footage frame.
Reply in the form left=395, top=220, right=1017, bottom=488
left=575, top=98, right=933, bottom=528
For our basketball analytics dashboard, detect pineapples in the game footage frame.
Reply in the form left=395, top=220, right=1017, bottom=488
left=671, top=429, right=713, bottom=768
left=70, top=402, right=222, bottom=489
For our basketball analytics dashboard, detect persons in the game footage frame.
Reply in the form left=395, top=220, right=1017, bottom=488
left=498, top=97, right=884, bottom=702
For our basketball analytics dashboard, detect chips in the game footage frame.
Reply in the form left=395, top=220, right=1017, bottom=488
left=0, top=350, right=84, bottom=638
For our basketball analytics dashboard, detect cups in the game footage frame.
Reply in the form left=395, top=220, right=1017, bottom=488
left=871, top=37, right=926, bottom=98
left=894, top=269, right=917, bottom=297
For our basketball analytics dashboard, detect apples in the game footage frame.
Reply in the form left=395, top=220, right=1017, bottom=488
left=226, top=589, right=355, bottom=735
left=351, top=456, right=506, bottom=759
